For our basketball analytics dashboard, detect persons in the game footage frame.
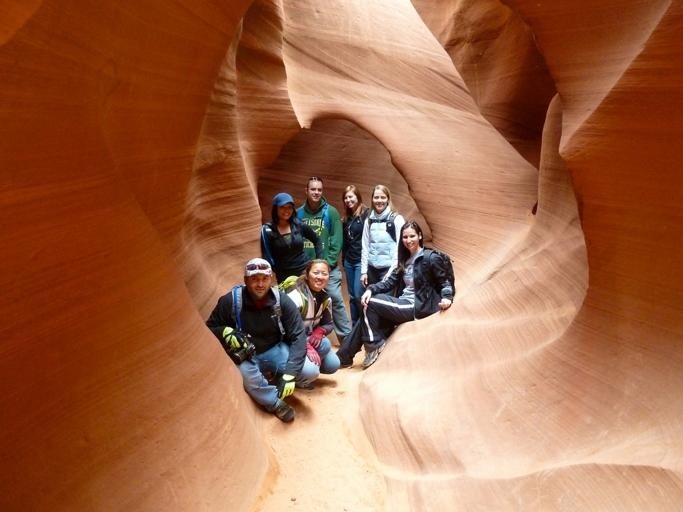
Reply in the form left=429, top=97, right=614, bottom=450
left=204, top=258, right=320, bottom=423
left=260, top=193, right=324, bottom=285
left=359, top=184, right=408, bottom=298
left=334, top=219, right=457, bottom=371
left=284, top=258, right=342, bottom=375
left=336, top=184, right=370, bottom=329
left=296, top=176, right=352, bottom=346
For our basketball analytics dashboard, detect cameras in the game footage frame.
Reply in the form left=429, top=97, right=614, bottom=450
left=232, top=335, right=257, bottom=361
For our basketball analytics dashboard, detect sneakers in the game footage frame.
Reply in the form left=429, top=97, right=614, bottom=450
left=275, top=401, right=295, bottom=423
left=361, top=342, right=385, bottom=368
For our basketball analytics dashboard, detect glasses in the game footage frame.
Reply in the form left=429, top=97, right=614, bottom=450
left=245, top=264, right=269, bottom=270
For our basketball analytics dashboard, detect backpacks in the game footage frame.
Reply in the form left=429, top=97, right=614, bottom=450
left=421, top=246, right=455, bottom=299
left=278, top=275, right=300, bottom=293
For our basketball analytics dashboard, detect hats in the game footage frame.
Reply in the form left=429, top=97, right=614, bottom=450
left=272, top=193, right=295, bottom=207
left=244, top=257, right=271, bottom=276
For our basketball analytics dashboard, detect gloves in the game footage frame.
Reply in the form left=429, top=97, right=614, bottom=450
left=278, top=375, right=296, bottom=400
left=306, top=327, right=326, bottom=366
left=223, top=326, right=249, bottom=349
